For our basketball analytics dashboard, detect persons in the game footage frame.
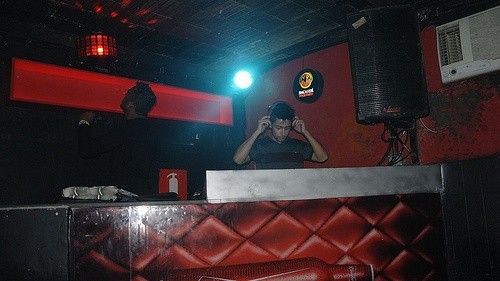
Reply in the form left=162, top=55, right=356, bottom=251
left=233, top=101, right=328, bottom=169
left=76, top=80, right=158, bottom=195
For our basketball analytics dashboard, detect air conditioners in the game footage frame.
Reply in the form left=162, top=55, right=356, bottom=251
left=435, top=5, right=500, bottom=85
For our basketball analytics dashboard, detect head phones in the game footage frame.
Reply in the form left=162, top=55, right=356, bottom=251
left=263, top=100, right=296, bottom=131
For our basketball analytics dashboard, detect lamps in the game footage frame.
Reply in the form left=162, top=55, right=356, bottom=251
left=75, top=32, right=119, bottom=67
left=234, top=63, right=259, bottom=89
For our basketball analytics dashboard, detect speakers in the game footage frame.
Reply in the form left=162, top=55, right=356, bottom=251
left=345, top=3, right=429, bottom=124
